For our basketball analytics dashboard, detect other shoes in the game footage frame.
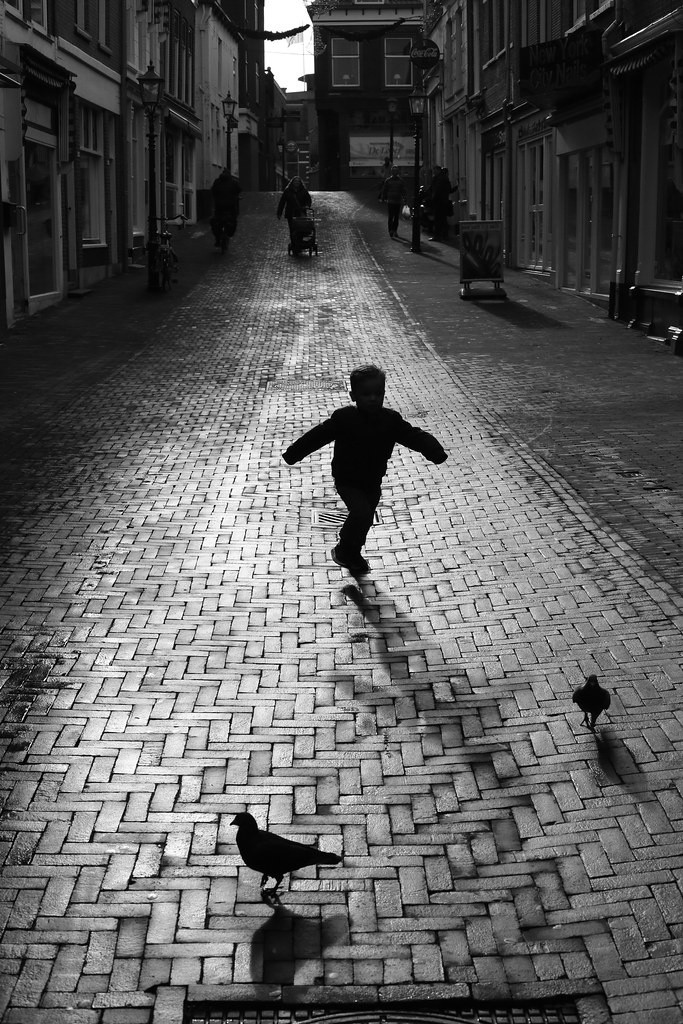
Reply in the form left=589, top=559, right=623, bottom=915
left=428, top=234, right=441, bottom=241
left=331, top=544, right=369, bottom=575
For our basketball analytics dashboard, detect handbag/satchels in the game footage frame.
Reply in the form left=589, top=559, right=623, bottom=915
left=446, top=200, right=454, bottom=217
left=402, top=205, right=410, bottom=218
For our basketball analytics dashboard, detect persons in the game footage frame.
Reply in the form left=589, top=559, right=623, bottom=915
left=282, top=365, right=447, bottom=577
left=382, top=165, right=408, bottom=237
left=421, top=166, right=458, bottom=242
left=210, top=167, right=242, bottom=249
left=277, top=176, right=312, bottom=255
left=378, top=157, right=394, bottom=199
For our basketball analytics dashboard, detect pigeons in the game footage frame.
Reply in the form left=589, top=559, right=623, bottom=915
left=572, top=675, right=610, bottom=727
left=230, top=812, right=345, bottom=896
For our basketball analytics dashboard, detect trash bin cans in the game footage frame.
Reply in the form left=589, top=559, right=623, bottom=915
left=196, top=189, right=214, bottom=220
